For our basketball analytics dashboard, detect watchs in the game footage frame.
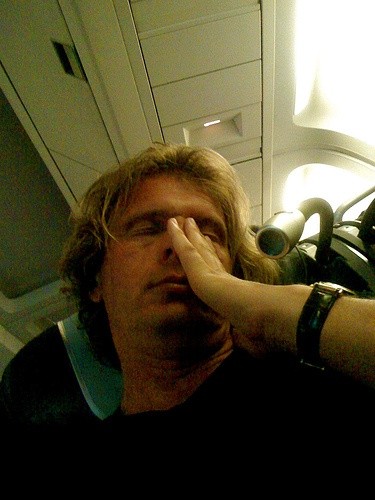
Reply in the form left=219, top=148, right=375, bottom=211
left=294, top=281, right=358, bottom=376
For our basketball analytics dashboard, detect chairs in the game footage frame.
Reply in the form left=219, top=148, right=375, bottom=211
left=1, top=233, right=369, bottom=444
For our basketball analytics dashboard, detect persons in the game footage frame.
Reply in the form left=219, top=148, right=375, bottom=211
left=31, top=141, right=352, bottom=499
left=163, top=218, right=375, bottom=373
